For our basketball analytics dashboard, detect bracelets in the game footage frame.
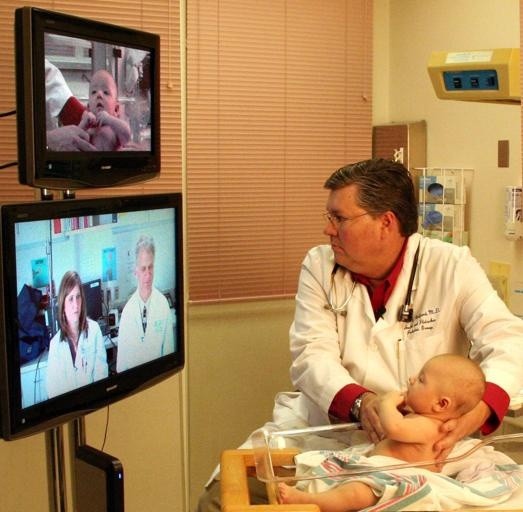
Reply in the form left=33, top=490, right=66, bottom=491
left=350, top=392, right=372, bottom=421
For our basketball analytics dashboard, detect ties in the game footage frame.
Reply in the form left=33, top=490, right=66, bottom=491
left=142, top=306, right=146, bottom=332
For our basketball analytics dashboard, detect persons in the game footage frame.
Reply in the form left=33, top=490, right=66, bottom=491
left=116, top=235, right=176, bottom=373
left=198, top=154, right=523, bottom=511
left=47, top=270, right=109, bottom=400
left=276, top=353, right=485, bottom=511
left=45, top=52, right=132, bottom=152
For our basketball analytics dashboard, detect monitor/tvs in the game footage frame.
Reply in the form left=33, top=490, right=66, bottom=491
left=0, top=192, right=185, bottom=442
left=14, top=5, right=161, bottom=190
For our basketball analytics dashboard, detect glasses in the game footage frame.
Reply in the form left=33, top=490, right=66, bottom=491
left=323, top=211, right=370, bottom=225
